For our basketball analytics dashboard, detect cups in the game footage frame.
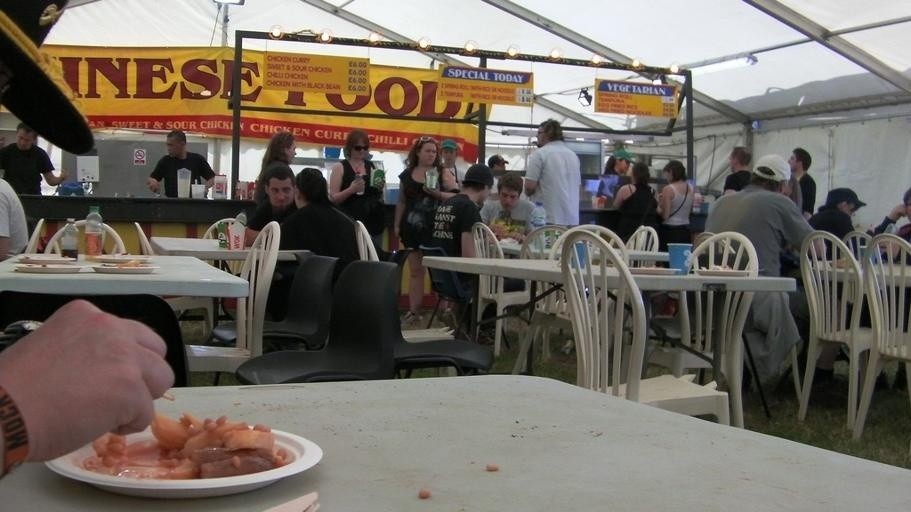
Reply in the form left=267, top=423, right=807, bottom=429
left=569, top=241, right=587, bottom=268
left=667, top=243, right=694, bottom=276
left=370, top=167, right=384, bottom=189
left=177, top=167, right=190, bottom=198
left=192, top=184, right=205, bottom=199
left=859, top=246, right=877, bottom=267
left=213, top=175, right=228, bottom=202
left=82, top=183, right=94, bottom=196
left=425, top=167, right=437, bottom=189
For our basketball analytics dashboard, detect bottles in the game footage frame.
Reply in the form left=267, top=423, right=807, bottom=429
left=355, top=168, right=364, bottom=195
left=60, top=219, right=79, bottom=259
left=590, top=194, right=608, bottom=208
left=692, top=191, right=702, bottom=213
left=85, top=205, right=104, bottom=256
left=234, top=179, right=254, bottom=201
left=530, top=201, right=548, bottom=249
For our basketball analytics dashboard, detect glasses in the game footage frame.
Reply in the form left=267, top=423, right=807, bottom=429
left=352, top=145, right=369, bottom=152
left=417, top=136, right=439, bottom=148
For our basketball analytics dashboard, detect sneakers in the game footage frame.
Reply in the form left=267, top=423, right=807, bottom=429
left=400, top=307, right=496, bottom=346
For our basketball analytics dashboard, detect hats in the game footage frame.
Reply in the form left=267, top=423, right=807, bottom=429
left=0, top=0, right=94, bottom=154
left=826, top=187, right=866, bottom=212
left=752, top=154, right=792, bottom=182
left=613, top=149, right=637, bottom=165
left=440, top=139, right=458, bottom=150
left=461, top=164, right=493, bottom=185
left=488, top=155, right=509, bottom=166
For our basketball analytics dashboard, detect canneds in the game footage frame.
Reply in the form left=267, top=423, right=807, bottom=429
left=544, top=229, right=562, bottom=249
left=217, top=222, right=229, bottom=247
left=235, top=182, right=249, bottom=200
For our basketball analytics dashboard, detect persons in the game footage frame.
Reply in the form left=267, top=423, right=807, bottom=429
left=232, top=119, right=582, bottom=326
left=592, top=119, right=911, bottom=264
left=1, top=120, right=70, bottom=197
left=0, top=177, right=30, bottom=264
left=0, top=298, right=179, bottom=485
left=147, top=127, right=216, bottom=199
left=707, top=152, right=855, bottom=401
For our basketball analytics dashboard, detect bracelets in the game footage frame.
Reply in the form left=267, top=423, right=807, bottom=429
left=0, top=385, right=30, bottom=477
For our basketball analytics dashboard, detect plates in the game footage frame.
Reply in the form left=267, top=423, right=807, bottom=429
left=46, top=423, right=321, bottom=499
left=19, top=255, right=158, bottom=276
left=627, top=267, right=681, bottom=275
left=694, top=270, right=751, bottom=276
